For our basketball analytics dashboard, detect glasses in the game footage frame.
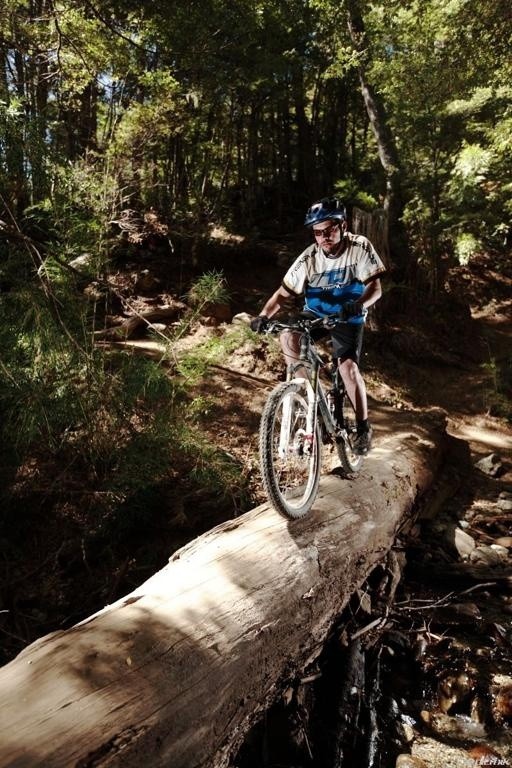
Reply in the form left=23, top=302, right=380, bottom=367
left=312, top=223, right=339, bottom=236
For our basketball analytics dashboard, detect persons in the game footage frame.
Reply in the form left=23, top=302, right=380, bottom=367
left=250, top=195, right=386, bottom=455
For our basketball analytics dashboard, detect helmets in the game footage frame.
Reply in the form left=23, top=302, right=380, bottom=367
left=305, top=197, right=347, bottom=226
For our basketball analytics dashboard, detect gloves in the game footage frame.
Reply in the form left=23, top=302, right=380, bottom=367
left=340, top=302, right=364, bottom=322
left=249, top=316, right=268, bottom=334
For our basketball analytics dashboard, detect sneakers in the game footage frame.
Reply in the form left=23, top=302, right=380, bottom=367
left=353, top=428, right=374, bottom=455
left=317, top=415, right=331, bottom=444
